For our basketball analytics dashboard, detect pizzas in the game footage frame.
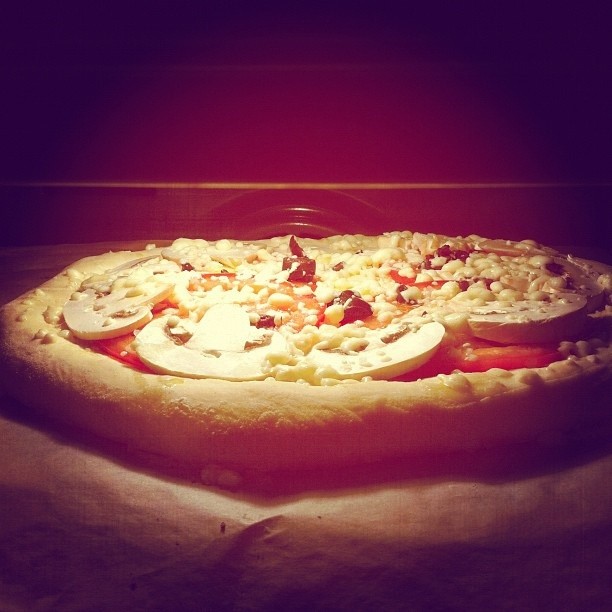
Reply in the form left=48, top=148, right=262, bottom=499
left=2, top=230, right=612, bottom=497
